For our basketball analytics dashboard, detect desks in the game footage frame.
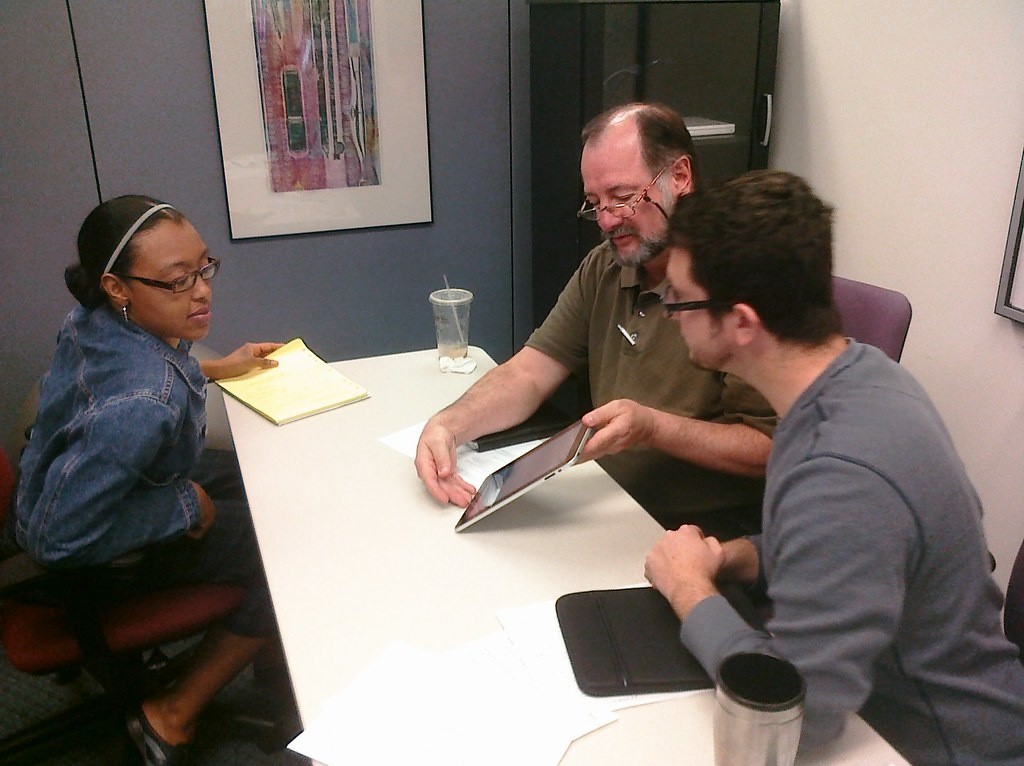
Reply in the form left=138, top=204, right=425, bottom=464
left=224, top=347, right=914, bottom=766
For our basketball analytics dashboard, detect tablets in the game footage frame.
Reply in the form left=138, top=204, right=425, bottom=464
left=455, top=419, right=593, bottom=532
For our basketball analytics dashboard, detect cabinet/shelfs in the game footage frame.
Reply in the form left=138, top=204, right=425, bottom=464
left=526, top=0, right=781, bottom=423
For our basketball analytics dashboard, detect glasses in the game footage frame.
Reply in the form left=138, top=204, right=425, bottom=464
left=577, top=166, right=667, bottom=221
left=663, top=285, right=710, bottom=318
left=114, top=257, right=220, bottom=293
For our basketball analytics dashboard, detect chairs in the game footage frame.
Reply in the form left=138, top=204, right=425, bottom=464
left=0, top=445, right=284, bottom=766
left=830, top=276, right=912, bottom=362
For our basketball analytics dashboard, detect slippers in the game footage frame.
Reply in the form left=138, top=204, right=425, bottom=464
left=125, top=703, right=195, bottom=766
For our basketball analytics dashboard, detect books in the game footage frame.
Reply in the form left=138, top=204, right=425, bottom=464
left=215, top=338, right=373, bottom=427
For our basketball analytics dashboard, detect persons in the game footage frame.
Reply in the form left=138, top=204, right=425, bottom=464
left=14, top=194, right=287, bottom=766
left=414, top=102, right=776, bottom=543
left=644, top=171, right=1023, bottom=766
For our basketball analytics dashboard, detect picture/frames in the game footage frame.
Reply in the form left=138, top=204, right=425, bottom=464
left=202, top=0, right=434, bottom=242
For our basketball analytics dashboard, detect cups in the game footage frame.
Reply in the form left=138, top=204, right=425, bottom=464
left=428, top=289, right=473, bottom=360
left=713, top=651, right=806, bottom=766
